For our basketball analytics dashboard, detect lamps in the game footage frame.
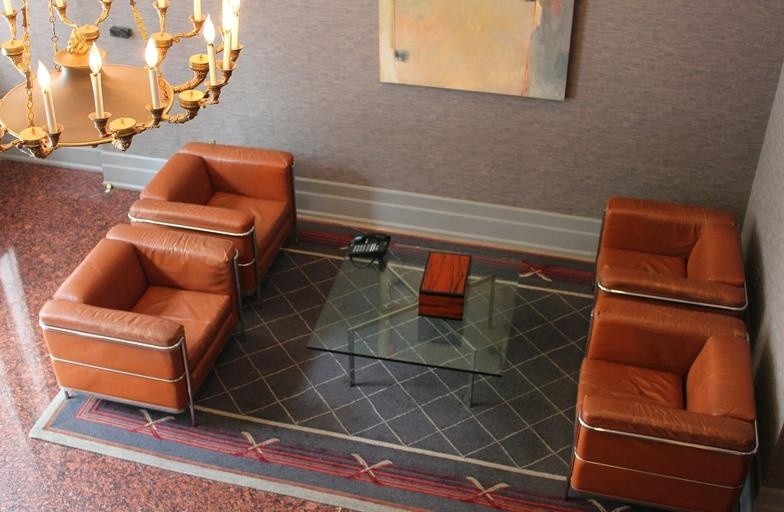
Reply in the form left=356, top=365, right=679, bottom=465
left=0, top=0, right=242, bottom=159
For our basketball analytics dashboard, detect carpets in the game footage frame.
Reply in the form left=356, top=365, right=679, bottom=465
left=26, top=213, right=674, bottom=511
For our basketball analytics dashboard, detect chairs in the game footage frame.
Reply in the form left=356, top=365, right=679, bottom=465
left=126, top=144, right=296, bottom=311
left=562, top=295, right=759, bottom=510
left=593, top=196, right=752, bottom=317
left=37, top=223, right=247, bottom=427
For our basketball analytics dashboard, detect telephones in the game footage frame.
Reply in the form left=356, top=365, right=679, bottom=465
left=348, top=233, right=391, bottom=258
